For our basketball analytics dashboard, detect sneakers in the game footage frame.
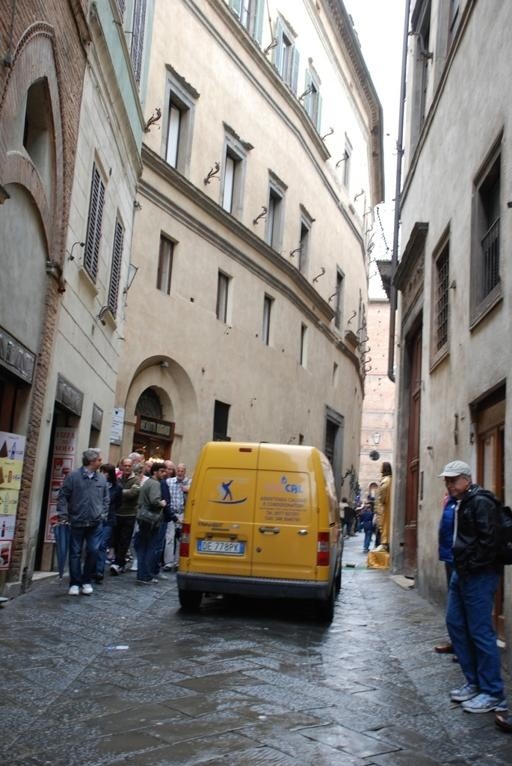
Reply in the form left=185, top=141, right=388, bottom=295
left=364, top=549, right=369, bottom=553
left=136, top=577, right=159, bottom=585
left=449, top=681, right=481, bottom=702
left=130, top=559, right=141, bottom=572
left=163, top=563, right=174, bottom=571
left=68, top=585, right=80, bottom=596
left=81, top=584, right=94, bottom=595
left=156, top=573, right=169, bottom=581
left=460, top=690, right=508, bottom=714
left=89, top=589, right=90, bottom=590
left=111, top=563, right=124, bottom=573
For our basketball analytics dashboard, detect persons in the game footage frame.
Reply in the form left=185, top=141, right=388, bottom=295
left=360, top=504, right=373, bottom=552
left=135, top=462, right=166, bottom=584
left=435, top=493, right=460, bottom=662
left=439, top=460, right=508, bottom=713
left=163, top=462, right=192, bottom=571
left=96, top=463, right=122, bottom=584
left=338, top=497, right=349, bottom=526
left=56, top=448, right=109, bottom=596
left=109, top=458, right=140, bottom=575
left=153, top=460, right=182, bottom=579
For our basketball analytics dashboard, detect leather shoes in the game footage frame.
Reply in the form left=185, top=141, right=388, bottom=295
left=451, top=655, right=460, bottom=662
left=434, top=645, right=452, bottom=651
left=495, top=710, right=512, bottom=733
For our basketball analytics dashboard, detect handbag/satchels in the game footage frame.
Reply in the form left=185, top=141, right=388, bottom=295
left=172, top=524, right=182, bottom=557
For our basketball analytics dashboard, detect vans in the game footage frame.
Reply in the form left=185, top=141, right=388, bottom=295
left=178, top=440, right=356, bottom=625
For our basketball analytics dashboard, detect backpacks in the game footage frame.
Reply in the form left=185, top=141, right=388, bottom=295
left=480, top=490, right=512, bottom=566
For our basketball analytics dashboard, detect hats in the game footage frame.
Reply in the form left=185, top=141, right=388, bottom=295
left=436, top=460, right=474, bottom=478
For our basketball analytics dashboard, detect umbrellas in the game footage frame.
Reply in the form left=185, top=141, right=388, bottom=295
left=52, top=522, right=70, bottom=581
